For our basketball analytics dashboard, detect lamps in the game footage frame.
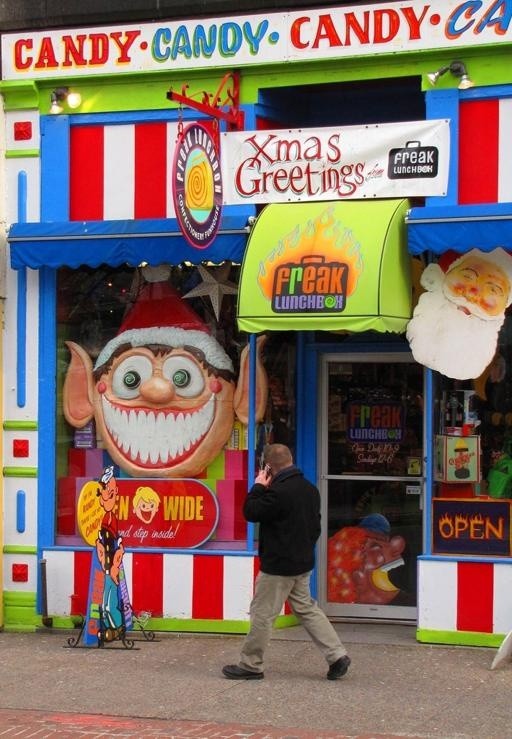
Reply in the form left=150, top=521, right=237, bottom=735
left=425, top=62, right=473, bottom=90
left=49, top=86, right=81, bottom=115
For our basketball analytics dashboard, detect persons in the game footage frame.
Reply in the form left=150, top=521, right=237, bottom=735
left=222, top=443, right=351, bottom=681
left=62, top=260, right=271, bottom=479
left=405, top=245, right=511, bottom=382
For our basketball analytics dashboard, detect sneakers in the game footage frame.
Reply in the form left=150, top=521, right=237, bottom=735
left=327, top=655, right=351, bottom=679
left=222, top=665, right=264, bottom=680
